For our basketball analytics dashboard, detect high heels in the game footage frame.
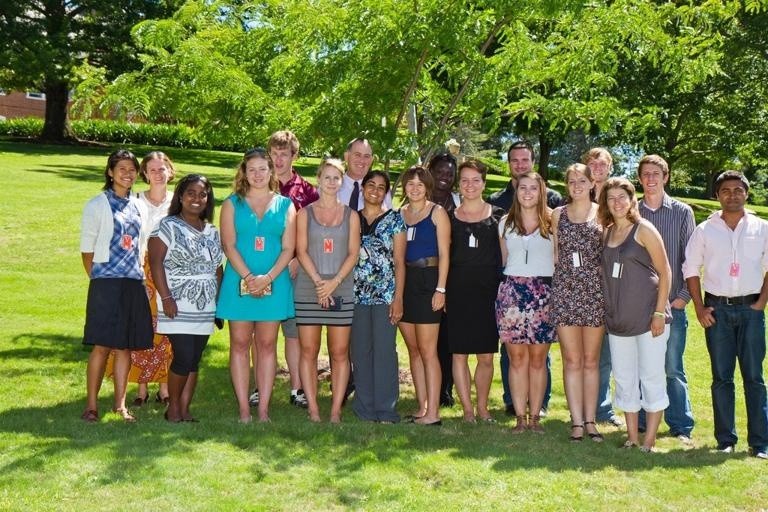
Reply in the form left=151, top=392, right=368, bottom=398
left=155, top=391, right=170, bottom=407
left=133, top=390, right=150, bottom=406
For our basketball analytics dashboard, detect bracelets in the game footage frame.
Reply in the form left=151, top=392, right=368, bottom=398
left=160, top=295, right=173, bottom=300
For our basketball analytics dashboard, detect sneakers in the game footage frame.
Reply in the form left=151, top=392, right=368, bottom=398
left=249, top=389, right=261, bottom=407
left=288, top=389, right=309, bottom=409
left=675, top=430, right=768, bottom=459
left=439, top=386, right=457, bottom=408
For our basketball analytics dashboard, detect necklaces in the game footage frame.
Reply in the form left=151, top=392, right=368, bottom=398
left=180, top=213, right=203, bottom=230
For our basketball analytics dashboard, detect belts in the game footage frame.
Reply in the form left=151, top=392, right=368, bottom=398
left=703, top=290, right=762, bottom=306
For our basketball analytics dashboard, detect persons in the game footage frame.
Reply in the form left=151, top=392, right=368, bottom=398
left=682, top=169, right=768, bottom=461
left=78, top=149, right=154, bottom=421
left=147, top=172, right=226, bottom=421
left=214, top=125, right=698, bottom=453
left=107, top=150, right=185, bottom=407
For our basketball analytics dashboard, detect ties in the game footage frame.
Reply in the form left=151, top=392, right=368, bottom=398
left=348, top=181, right=361, bottom=211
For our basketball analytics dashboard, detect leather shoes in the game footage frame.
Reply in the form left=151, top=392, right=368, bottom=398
left=504, top=403, right=518, bottom=415
left=179, top=412, right=200, bottom=423
left=605, top=412, right=624, bottom=428
left=164, top=410, right=184, bottom=425
left=405, top=414, right=443, bottom=427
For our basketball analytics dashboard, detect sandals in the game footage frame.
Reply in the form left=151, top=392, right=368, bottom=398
left=527, top=415, right=546, bottom=434
left=81, top=407, right=100, bottom=425
left=460, top=410, right=498, bottom=426
left=112, top=406, right=136, bottom=422
left=568, top=425, right=586, bottom=444
left=584, top=421, right=605, bottom=444
left=510, top=415, right=529, bottom=435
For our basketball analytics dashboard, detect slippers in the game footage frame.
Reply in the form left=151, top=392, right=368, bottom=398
left=620, top=440, right=656, bottom=455
